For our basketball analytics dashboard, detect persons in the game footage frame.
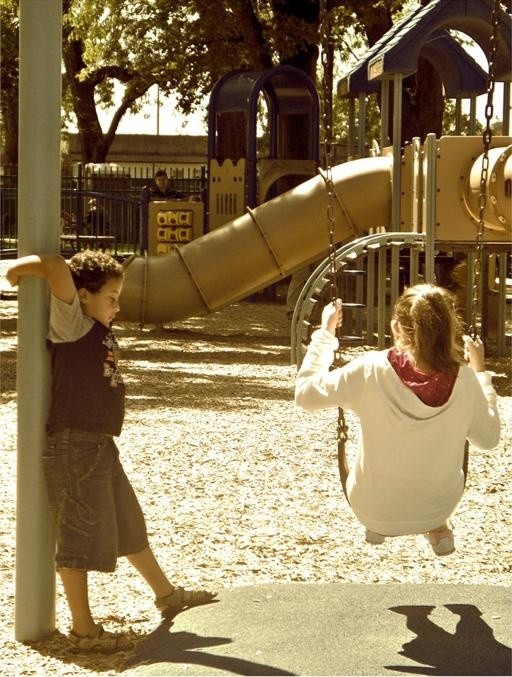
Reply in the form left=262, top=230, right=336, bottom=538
left=5, top=249, right=218, bottom=653
left=294, top=283, right=501, bottom=555
left=87, top=198, right=112, bottom=248
left=148, top=170, right=186, bottom=201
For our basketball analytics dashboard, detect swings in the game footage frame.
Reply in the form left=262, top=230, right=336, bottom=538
left=317, top=1, right=504, bottom=538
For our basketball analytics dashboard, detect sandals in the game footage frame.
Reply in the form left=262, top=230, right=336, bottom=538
left=68, top=625, right=134, bottom=654
left=154, top=587, right=218, bottom=619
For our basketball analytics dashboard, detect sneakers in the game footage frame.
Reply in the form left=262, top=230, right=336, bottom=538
left=426, top=529, right=455, bottom=558
left=365, top=530, right=385, bottom=546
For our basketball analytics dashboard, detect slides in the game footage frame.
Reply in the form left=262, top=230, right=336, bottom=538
left=110, top=142, right=412, bottom=326
